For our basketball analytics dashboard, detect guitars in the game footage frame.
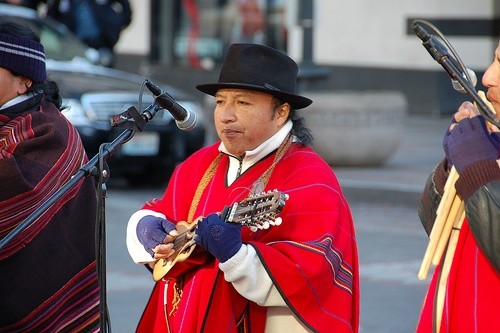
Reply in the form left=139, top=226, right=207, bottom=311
left=153, top=187, right=291, bottom=284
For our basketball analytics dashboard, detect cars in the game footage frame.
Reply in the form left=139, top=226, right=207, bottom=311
left=0, top=3, right=207, bottom=190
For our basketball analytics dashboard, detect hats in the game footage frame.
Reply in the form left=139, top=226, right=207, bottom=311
left=0, top=32, right=47, bottom=83
left=195, top=42, right=313, bottom=111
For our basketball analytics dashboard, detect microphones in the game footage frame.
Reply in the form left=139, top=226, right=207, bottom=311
left=412, top=24, right=478, bottom=93
left=144, top=78, right=198, bottom=131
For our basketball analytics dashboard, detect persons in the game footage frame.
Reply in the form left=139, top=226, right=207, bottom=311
left=416, top=40, right=500, bottom=333
left=0, top=21, right=110, bottom=333
left=125, top=44, right=360, bottom=333
